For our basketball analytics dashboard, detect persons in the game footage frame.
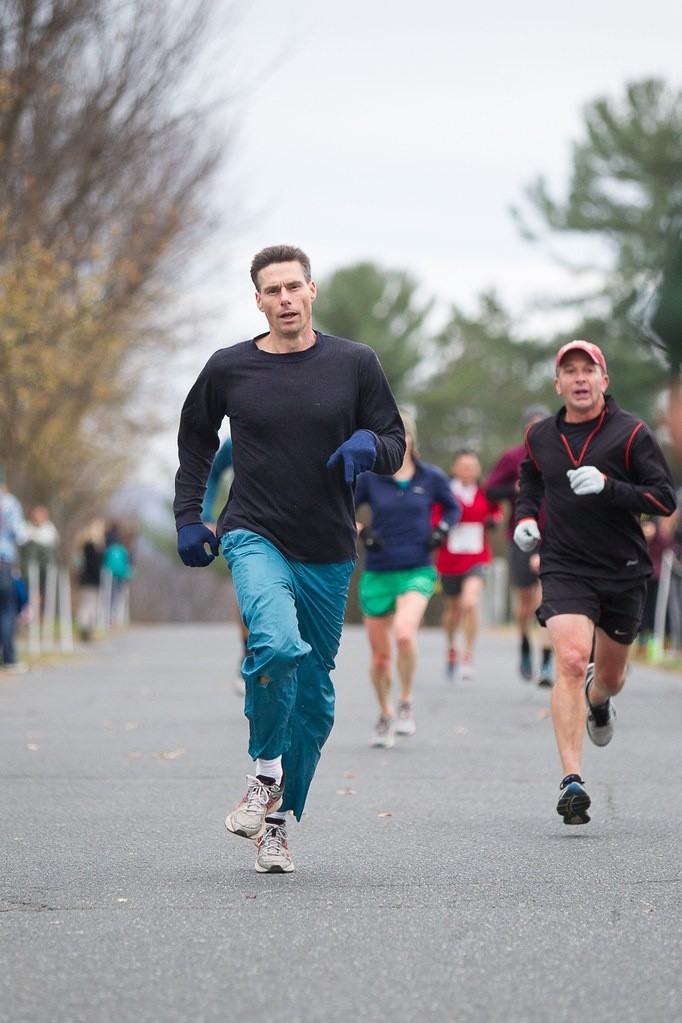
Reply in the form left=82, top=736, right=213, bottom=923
left=0, top=484, right=140, bottom=672
left=511, top=340, right=677, bottom=826
left=172, top=245, right=409, bottom=873
left=209, top=398, right=682, bottom=746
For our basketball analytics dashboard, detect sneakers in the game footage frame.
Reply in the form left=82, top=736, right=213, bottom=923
left=255, top=809, right=295, bottom=873
left=557, top=772, right=590, bottom=824
left=367, top=714, right=396, bottom=749
left=395, top=697, right=415, bottom=735
left=583, top=660, right=616, bottom=746
left=224, top=766, right=285, bottom=837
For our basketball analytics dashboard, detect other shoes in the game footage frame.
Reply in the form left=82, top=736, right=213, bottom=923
left=540, top=662, right=555, bottom=686
left=446, top=649, right=457, bottom=682
left=520, top=653, right=533, bottom=679
left=461, top=661, right=476, bottom=681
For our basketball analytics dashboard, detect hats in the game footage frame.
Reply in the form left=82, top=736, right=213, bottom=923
left=555, top=340, right=607, bottom=375
left=522, top=403, right=552, bottom=426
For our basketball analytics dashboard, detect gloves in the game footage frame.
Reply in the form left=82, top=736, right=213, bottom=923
left=514, top=516, right=541, bottom=552
left=327, top=430, right=376, bottom=485
left=566, top=465, right=605, bottom=497
left=428, top=526, right=446, bottom=549
left=360, top=526, right=384, bottom=553
left=177, top=522, right=220, bottom=567
